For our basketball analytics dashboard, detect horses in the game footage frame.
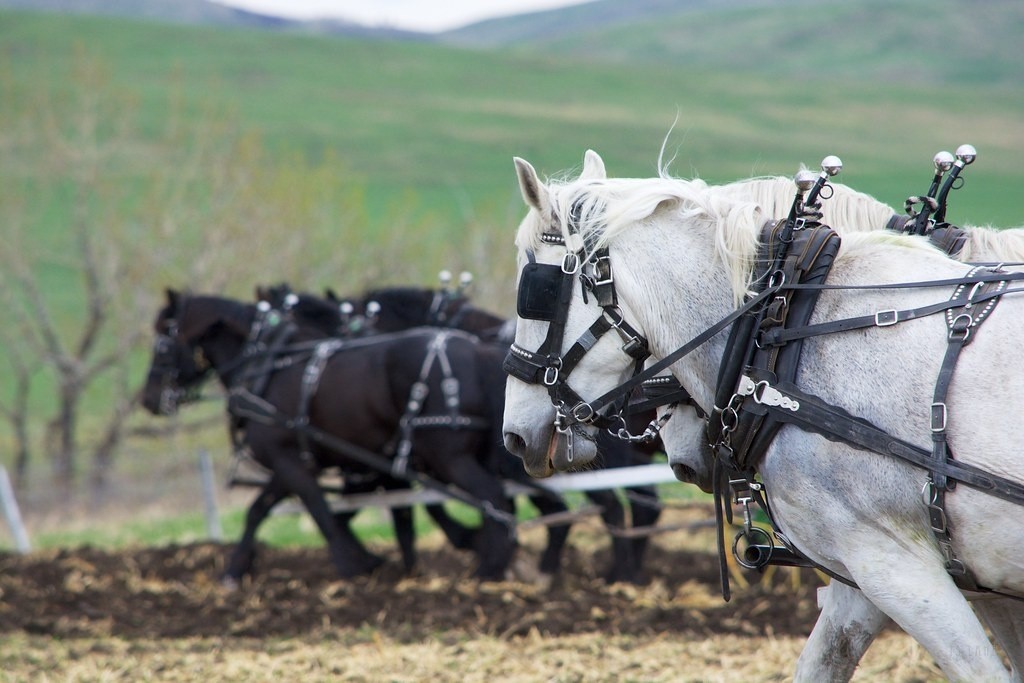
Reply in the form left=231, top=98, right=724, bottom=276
left=498, top=143, right=1024, bottom=683
left=139, top=280, right=666, bottom=590
